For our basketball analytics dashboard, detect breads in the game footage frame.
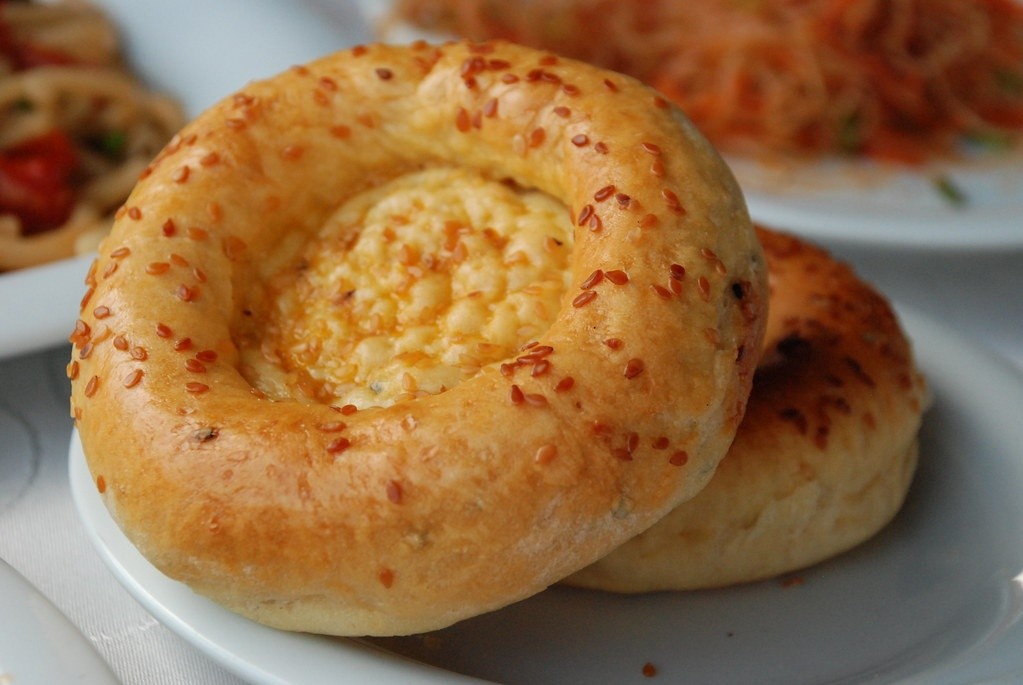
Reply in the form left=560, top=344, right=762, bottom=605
left=65, top=40, right=771, bottom=635
left=555, top=220, right=932, bottom=593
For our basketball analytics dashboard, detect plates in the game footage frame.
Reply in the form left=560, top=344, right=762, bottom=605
left=359, top=1, right=1023, bottom=256
left=0, top=0, right=344, bottom=363
left=68, top=298, right=1023, bottom=685
